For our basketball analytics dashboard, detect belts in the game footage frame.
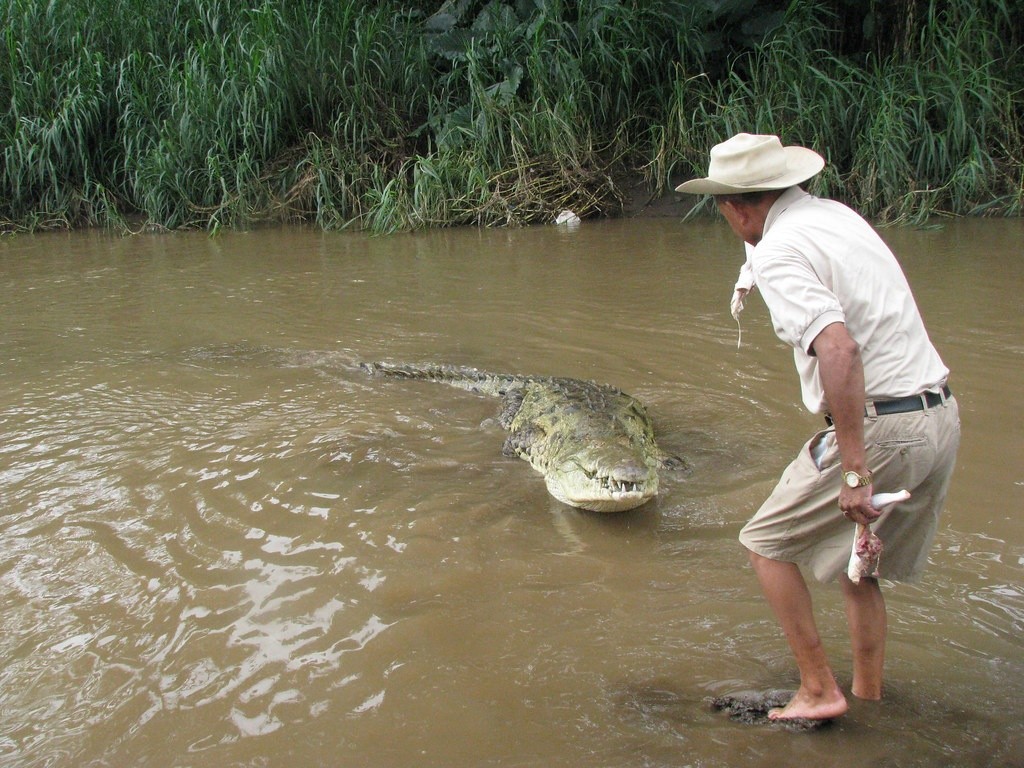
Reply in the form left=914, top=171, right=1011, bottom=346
left=824, top=386, right=952, bottom=426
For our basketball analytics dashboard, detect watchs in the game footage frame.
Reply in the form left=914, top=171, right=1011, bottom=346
left=839, top=469, right=874, bottom=489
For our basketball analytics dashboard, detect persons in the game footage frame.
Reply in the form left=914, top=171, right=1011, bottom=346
left=674, top=133, right=962, bottom=718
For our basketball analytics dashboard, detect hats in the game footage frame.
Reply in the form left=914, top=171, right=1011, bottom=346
left=674, top=132, right=824, bottom=194
